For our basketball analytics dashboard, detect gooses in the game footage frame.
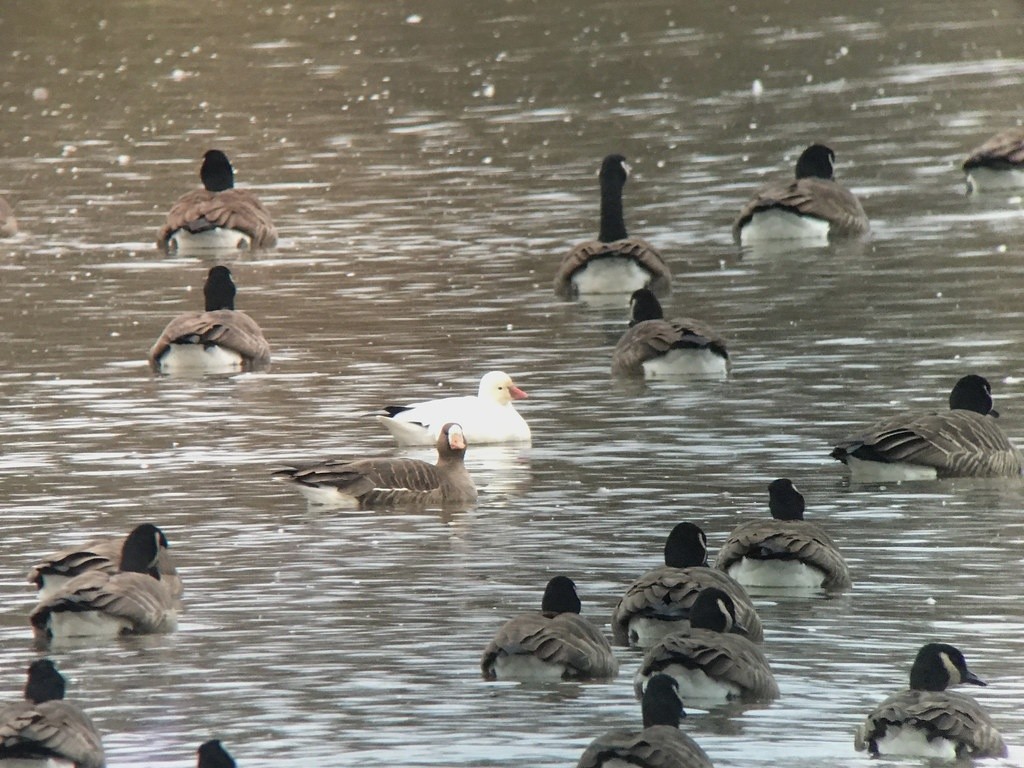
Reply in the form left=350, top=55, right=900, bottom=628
left=268, top=422, right=478, bottom=507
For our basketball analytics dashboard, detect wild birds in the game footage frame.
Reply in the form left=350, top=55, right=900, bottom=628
left=479, top=575, right=619, bottom=680
left=614, top=289, right=731, bottom=374
left=852, top=643, right=1010, bottom=760
left=633, top=586, right=783, bottom=701
left=158, top=148, right=278, bottom=248
left=552, top=152, right=671, bottom=290
left=961, top=125, right=1024, bottom=188
left=0, top=659, right=106, bottom=768
left=715, top=478, right=853, bottom=587
left=26, top=523, right=178, bottom=637
left=361, top=370, right=534, bottom=445
left=149, top=264, right=271, bottom=368
left=27, top=534, right=185, bottom=601
left=575, top=674, right=713, bottom=767
left=732, top=144, right=870, bottom=239
left=611, top=518, right=764, bottom=645
left=829, top=375, right=1023, bottom=477
